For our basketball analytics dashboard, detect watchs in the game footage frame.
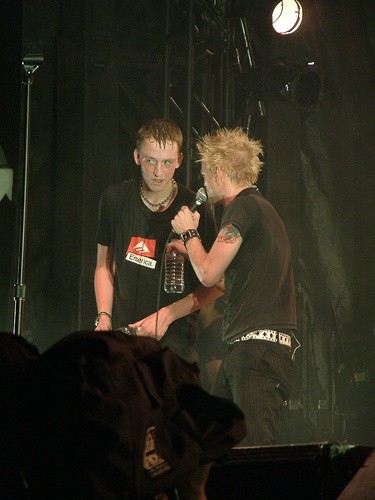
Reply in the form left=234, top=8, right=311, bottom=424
left=179, top=229, right=201, bottom=250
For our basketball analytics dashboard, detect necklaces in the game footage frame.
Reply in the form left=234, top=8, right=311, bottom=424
left=139, top=174, right=175, bottom=207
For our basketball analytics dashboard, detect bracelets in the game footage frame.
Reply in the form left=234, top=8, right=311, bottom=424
left=95, top=311, right=111, bottom=326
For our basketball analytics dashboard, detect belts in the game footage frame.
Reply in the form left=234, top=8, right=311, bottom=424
left=226, top=329, right=292, bottom=347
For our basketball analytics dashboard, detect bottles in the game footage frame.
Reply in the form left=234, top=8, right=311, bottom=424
left=164, top=239, right=184, bottom=293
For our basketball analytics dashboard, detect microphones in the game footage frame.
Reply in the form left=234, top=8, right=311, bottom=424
left=172, top=186, right=207, bottom=232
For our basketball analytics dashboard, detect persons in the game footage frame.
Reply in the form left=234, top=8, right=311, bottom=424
left=93, top=117, right=225, bottom=377
left=171, top=127, right=296, bottom=446
left=0, top=330, right=375, bottom=500
